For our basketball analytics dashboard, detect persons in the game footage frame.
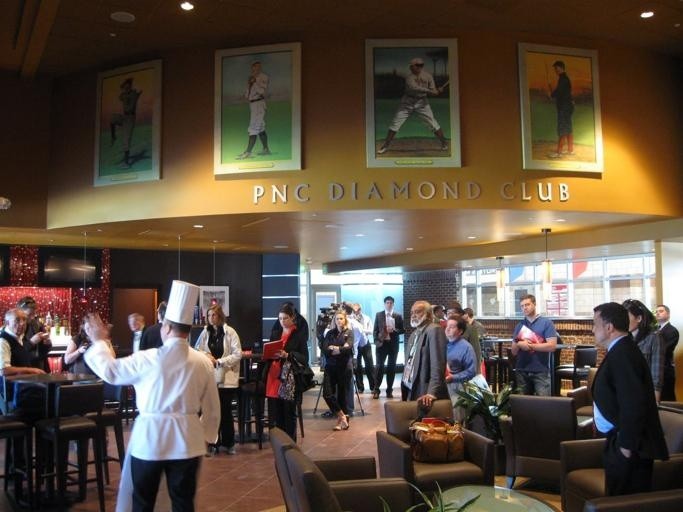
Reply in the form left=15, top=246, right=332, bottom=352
left=655, top=304, right=679, bottom=401
left=109, top=78, right=142, bottom=168
left=1, top=309, right=46, bottom=412
left=17, top=297, right=52, bottom=378
left=590, top=302, right=670, bottom=496
left=234, top=58, right=270, bottom=159
left=195, top=304, right=242, bottom=455
left=375, top=58, right=449, bottom=154
left=84, top=280, right=221, bottom=512
left=262, top=306, right=309, bottom=443
left=622, top=300, right=666, bottom=407
left=140, top=299, right=168, bottom=351
left=545, top=61, right=576, bottom=158
left=65, top=321, right=116, bottom=380
left=511, top=294, right=559, bottom=397
left=322, top=296, right=496, bottom=431
left=128, top=312, right=146, bottom=354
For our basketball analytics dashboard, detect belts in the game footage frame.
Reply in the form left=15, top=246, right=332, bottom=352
left=250, top=98, right=265, bottom=103
left=409, top=95, right=426, bottom=101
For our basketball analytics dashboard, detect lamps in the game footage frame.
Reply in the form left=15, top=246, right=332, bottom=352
left=496, top=256, right=505, bottom=288
left=541, top=229, right=552, bottom=284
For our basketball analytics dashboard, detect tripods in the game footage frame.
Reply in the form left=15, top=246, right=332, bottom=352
left=313, top=375, right=365, bottom=416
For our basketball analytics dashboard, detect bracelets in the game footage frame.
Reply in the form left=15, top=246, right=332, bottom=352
left=77, top=349, right=81, bottom=354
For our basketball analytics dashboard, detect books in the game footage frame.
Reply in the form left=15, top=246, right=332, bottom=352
left=263, top=340, right=283, bottom=361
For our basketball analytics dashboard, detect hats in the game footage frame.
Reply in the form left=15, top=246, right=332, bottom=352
left=552, top=61, right=565, bottom=67
left=409, top=58, right=425, bottom=66
left=165, top=280, right=200, bottom=326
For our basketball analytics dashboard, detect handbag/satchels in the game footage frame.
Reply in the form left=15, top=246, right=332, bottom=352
left=214, top=366, right=226, bottom=384
left=412, top=416, right=466, bottom=465
left=277, top=363, right=295, bottom=401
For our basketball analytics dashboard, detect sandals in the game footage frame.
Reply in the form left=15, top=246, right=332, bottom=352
left=333, top=416, right=349, bottom=431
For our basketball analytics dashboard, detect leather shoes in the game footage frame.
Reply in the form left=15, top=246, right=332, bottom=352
left=321, top=412, right=332, bottom=419
left=373, top=393, right=379, bottom=399
left=387, top=394, right=393, bottom=398
left=358, top=389, right=363, bottom=393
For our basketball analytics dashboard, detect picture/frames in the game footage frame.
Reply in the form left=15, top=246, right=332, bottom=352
left=92, top=58, right=163, bottom=188
left=364, top=37, right=462, bottom=168
left=199, top=285, right=230, bottom=318
left=213, top=41, right=303, bottom=176
left=516, top=40, right=605, bottom=175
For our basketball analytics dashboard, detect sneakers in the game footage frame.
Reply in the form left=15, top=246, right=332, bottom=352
left=550, top=151, right=574, bottom=158
left=236, top=152, right=251, bottom=159
left=209, top=445, right=236, bottom=455
left=258, top=148, right=271, bottom=155
left=120, top=163, right=129, bottom=168
left=377, top=145, right=388, bottom=154
left=441, top=143, right=447, bottom=150
left=110, top=137, right=116, bottom=146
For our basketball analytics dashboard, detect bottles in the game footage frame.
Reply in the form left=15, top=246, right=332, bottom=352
left=39, top=311, right=69, bottom=337
